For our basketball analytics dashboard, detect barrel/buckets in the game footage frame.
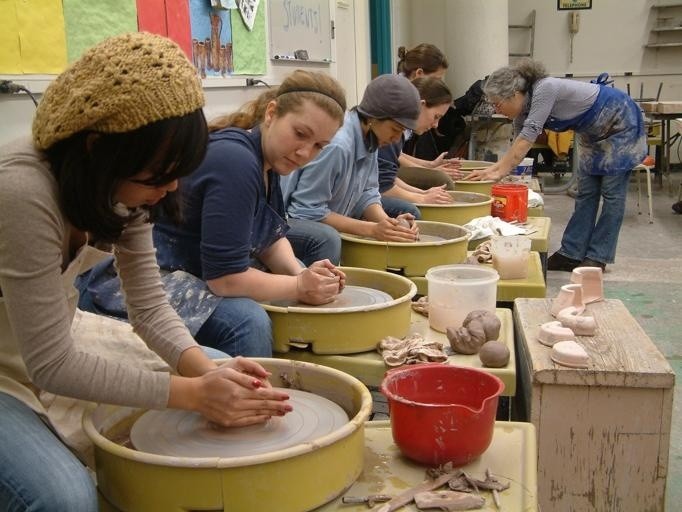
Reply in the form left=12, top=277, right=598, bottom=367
left=377, top=360, right=506, bottom=470
left=424, top=262, right=501, bottom=336
left=507, top=156, right=535, bottom=184
left=488, top=182, right=530, bottom=225
left=488, top=233, right=533, bottom=281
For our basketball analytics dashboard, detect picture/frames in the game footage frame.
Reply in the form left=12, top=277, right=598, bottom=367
left=558, top=0, right=592, bottom=10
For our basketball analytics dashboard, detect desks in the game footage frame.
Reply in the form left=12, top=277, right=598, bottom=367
left=645, top=111, right=682, bottom=198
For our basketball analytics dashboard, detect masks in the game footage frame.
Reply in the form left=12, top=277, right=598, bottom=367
left=364, top=127, right=379, bottom=155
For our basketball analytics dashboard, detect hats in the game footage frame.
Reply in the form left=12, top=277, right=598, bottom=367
left=356, top=73, right=422, bottom=131
left=32, top=31, right=206, bottom=153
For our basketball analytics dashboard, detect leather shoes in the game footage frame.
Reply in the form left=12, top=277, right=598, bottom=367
left=570, top=257, right=605, bottom=272
left=548, top=253, right=579, bottom=272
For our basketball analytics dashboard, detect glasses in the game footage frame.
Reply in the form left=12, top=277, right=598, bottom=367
left=490, top=97, right=507, bottom=110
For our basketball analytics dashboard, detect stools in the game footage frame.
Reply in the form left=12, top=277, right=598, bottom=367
left=630, top=163, right=656, bottom=225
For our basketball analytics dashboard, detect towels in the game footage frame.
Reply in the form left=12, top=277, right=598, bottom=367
left=376, top=334, right=451, bottom=370
left=412, top=295, right=430, bottom=317
left=470, top=239, right=493, bottom=263
left=460, top=214, right=536, bottom=242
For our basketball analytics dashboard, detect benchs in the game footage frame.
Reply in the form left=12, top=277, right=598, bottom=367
left=510, top=289, right=679, bottom=512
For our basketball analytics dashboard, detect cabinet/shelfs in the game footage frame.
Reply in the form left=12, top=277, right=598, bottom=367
left=644, top=1, right=682, bottom=49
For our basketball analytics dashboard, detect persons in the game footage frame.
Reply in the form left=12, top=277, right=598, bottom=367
left=378, top=76, right=454, bottom=221
left=75, top=68, right=346, bottom=357
left=461, top=56, right=649, bottom=273
left=0, top=29, right=296, bottom=512
left=280, top=73, right=423, bottom=268
left=396, top=42, right=466, bottom=182
